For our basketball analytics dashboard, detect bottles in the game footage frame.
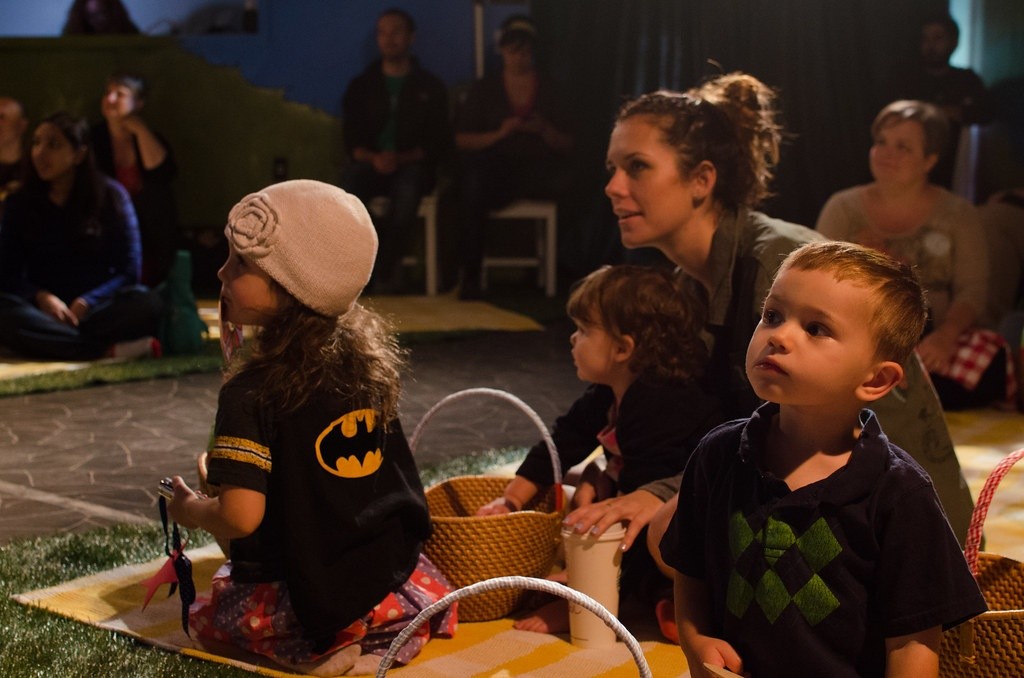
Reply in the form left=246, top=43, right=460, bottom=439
left=168, top=250, right=197, bottom=349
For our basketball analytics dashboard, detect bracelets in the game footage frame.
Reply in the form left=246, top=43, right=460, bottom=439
left=496, top=494, right=517, bottom=512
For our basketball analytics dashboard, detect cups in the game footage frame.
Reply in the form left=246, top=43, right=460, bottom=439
left=560, top=522, right=631, bottom=647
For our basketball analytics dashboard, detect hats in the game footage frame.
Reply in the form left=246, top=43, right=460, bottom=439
left=224, top=180, right=379, bottom=318
left=494, top=15, right=539, bottom=47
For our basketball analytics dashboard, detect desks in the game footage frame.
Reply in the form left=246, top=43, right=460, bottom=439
left=369, top=187, right=567, bottom=300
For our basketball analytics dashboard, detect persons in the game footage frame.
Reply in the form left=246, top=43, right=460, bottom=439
left=59, top=0, right=145, bottom=36
left=340, top=9, right=446, bottom=281
left=817, top=100, right=985, bottom=374
left=168, top=178, right=460, bottom=678
left=890, top=11, right=989, bottom=203
left=0, top=73, right=208, bottom=360
left=475, top=262, right=712, bottom=631
left=562, top=72, right=985, bottom=580
left=662, top=240, right=990, bottom=678
left=445, top=15, right=592, bottom=291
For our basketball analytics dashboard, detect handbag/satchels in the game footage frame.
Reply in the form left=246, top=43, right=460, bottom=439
left=935, top=451, right=1024, bottom=678
left=408, top=388, right=565, bottom=622
